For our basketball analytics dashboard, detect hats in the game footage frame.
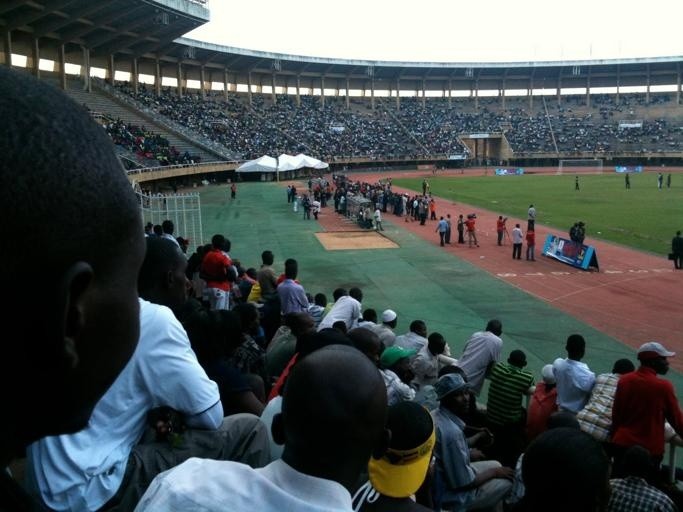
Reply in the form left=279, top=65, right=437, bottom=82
left=367, top=406, right=435, bottom=498
left=434, top=373, right=470, bottom=401
left=379, top=345, right=417, bottom=369
left=381, top=309, right=397, bottom=322
left=541, top=364, right=555, bottom=385
left=636, top=342, right=675, bottom=359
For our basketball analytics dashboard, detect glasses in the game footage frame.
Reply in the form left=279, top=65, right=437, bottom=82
left=372, top=444, right=418, bottom=465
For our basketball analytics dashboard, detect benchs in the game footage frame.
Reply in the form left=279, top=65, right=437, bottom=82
left=59, top=80, right=228, bottom=170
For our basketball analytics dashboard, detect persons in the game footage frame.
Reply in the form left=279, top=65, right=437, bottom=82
left=0, top=65, right=682, bottom=511
